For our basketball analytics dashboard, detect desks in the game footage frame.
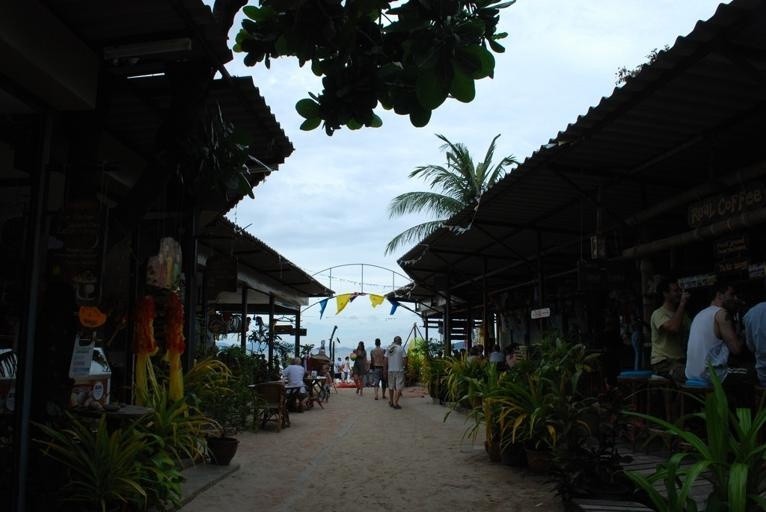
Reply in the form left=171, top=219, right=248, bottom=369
left=248, top=384, right=307, bottom=412
left=69, top=404, right=157, bottom=438
left=304, top=375, right=328, bottom=409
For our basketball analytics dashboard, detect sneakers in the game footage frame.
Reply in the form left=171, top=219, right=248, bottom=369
left=647, top=421, right=707, bottom=442
left=375, top=395, right=387, bottom=400
left=389, top=400, right=401, bottom=409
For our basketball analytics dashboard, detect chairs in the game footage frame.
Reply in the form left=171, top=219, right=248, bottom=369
left=248, top=383, right=291, bottom=432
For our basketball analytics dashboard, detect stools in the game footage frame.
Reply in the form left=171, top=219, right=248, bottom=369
left=614, top=369, right=766, bottom=464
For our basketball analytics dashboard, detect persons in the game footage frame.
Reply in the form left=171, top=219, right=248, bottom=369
left=436, top=351, right=443, bottom=358
left=280, top=355, right=308, bottom=408
left=455, top=334, right=537, bottom=369
left=334, top=336, right=410, bottom=411
left=648, top=279, right=762, bottom=394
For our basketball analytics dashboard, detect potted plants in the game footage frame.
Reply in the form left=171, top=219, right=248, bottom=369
left=419, top=337, right=610, bottom=469
left=193, top=369, right=258, bottom=465
left=405, top=348, right=417, bottom=374
